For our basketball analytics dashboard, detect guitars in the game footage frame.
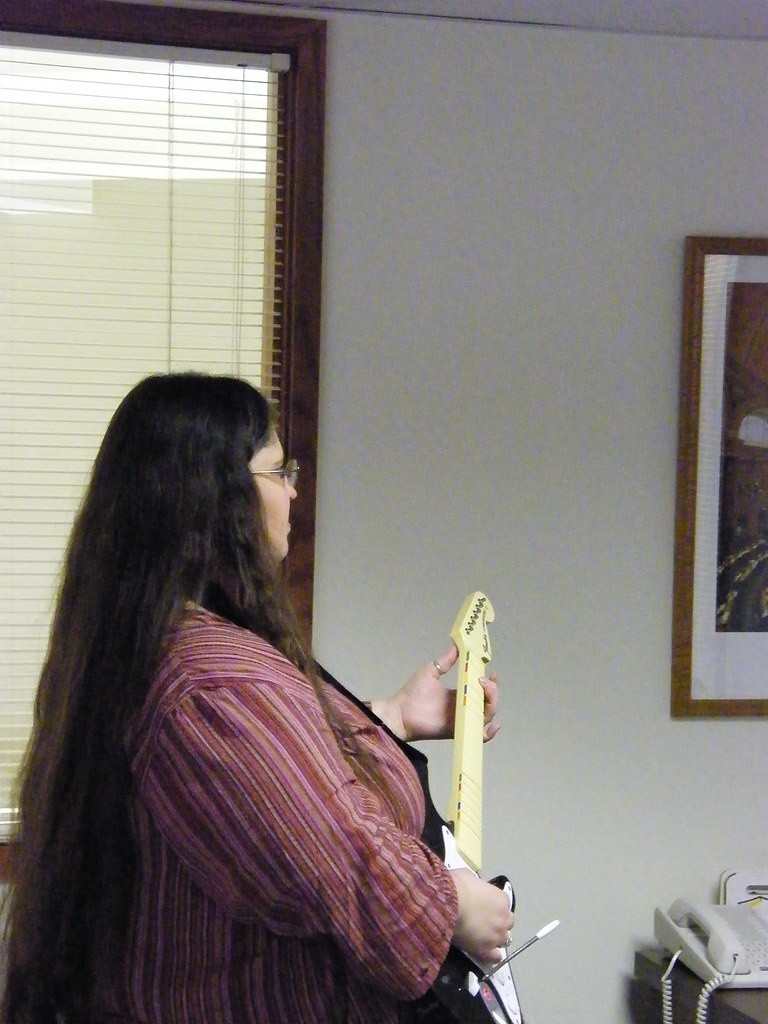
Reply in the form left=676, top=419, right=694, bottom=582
left=393, top=587, right=565, bottom=1024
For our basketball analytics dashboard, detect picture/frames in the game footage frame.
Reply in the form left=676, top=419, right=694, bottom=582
left=670, top=235, right=768, bottom=721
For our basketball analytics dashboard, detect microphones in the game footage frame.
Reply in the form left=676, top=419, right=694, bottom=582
left=481, top=919, right=560, bottom=981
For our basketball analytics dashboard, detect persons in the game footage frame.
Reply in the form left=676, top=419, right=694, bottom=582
left=1, top=373, right=516, bottom=1024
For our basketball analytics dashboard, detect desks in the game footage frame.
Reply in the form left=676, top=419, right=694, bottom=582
left=634, top=949, right=767, bottom=1024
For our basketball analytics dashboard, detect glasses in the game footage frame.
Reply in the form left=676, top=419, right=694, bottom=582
left=250, top=459, right=300, bottom=487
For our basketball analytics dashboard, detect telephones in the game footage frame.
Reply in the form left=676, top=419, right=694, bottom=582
left=652, top=897, right=768, bottom=989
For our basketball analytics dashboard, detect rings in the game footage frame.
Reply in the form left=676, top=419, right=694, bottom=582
left=432, top=660, right=444, bottom=675
left=499, top=930, right=513, bottom=948
